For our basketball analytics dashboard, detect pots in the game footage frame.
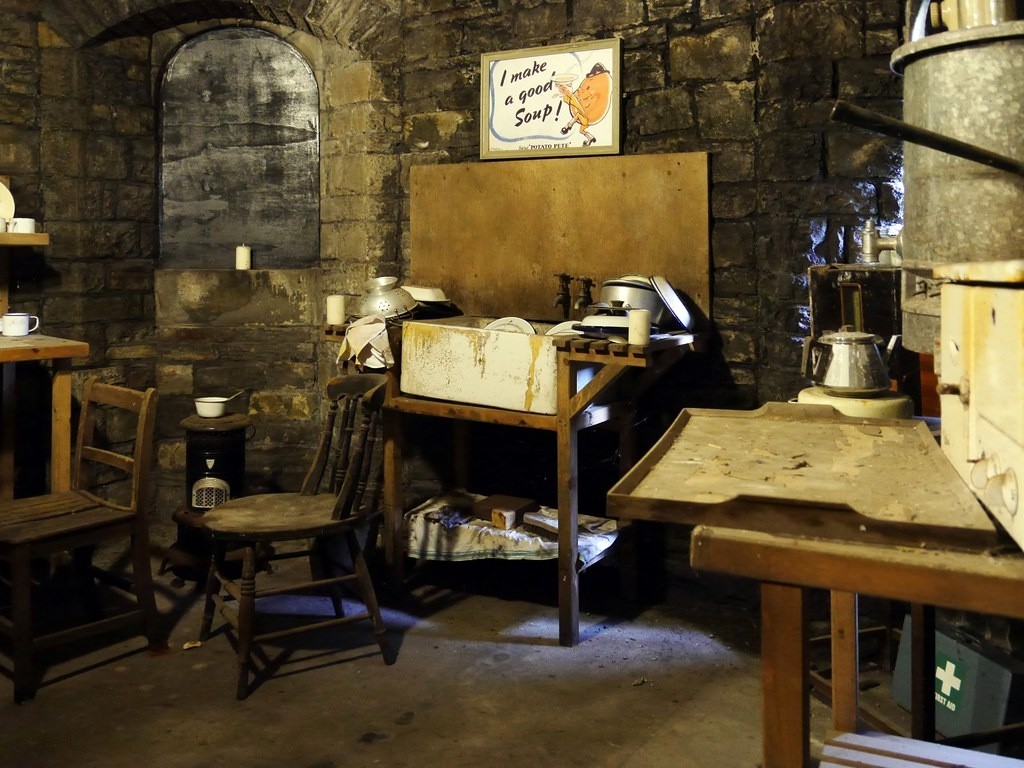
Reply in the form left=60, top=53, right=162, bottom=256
left=599, top=285, right=664, bottom=324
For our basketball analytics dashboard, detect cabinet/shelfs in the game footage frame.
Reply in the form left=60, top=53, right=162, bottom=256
left=0, top=175, right=90, bottom=597
left=690, top=259, right=1024, bottom=768
left=303, top=314, right=705, bottom=649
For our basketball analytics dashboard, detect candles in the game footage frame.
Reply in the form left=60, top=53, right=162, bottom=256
left=234, top=242, right=251, bottom=270
left=628, top=308, right=651, bottom=345
left=326, top=292, right=345, bottom=325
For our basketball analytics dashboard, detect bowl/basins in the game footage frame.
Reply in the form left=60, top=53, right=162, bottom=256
left=400, top=285, right=451, bottom=302
left=193, top=396, right=229, bottom=418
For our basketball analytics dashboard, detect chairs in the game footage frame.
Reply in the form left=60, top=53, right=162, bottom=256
left=200, top=373, right=396, bottom=701
left=0, top=375, right=164, bottom=708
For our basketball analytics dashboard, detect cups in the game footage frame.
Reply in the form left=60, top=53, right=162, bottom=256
left=1, top=313, right=39, bottom=337
left=8, top=218, right=35, bottom=233
left=0, top=218, right=6, bottom=232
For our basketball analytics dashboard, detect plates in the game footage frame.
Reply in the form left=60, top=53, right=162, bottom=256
left=545, top=321, right=584, bottom=336
left=571, top=323, right=660, bottom=339
left=0, top=181, right=15, bottom=222
left=649, top=275, right=695, bottom=334
left=483, top=317, right=535, bottom=334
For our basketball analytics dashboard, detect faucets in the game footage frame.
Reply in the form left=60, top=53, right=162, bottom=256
left=855, top=218, right=899, bottom=265
left=552, top=271, right=574, bottom=312
left=574, top=276, right=596, bottom=311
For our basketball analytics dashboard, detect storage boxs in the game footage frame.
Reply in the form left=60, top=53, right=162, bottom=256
left=472, top=493, right=538, bottom=530
left=890, top=612, right=1024, bottom=759
left=400, top=316, right=617, bottom=415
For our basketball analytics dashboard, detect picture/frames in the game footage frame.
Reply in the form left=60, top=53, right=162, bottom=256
left=479, top=37, right=621, bottom=161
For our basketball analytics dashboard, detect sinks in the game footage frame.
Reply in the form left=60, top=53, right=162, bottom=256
left=399, top=314, right=596, bottom=416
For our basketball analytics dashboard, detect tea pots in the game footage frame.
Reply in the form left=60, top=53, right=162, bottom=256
left=801, top=325, right=902, bottom=398
